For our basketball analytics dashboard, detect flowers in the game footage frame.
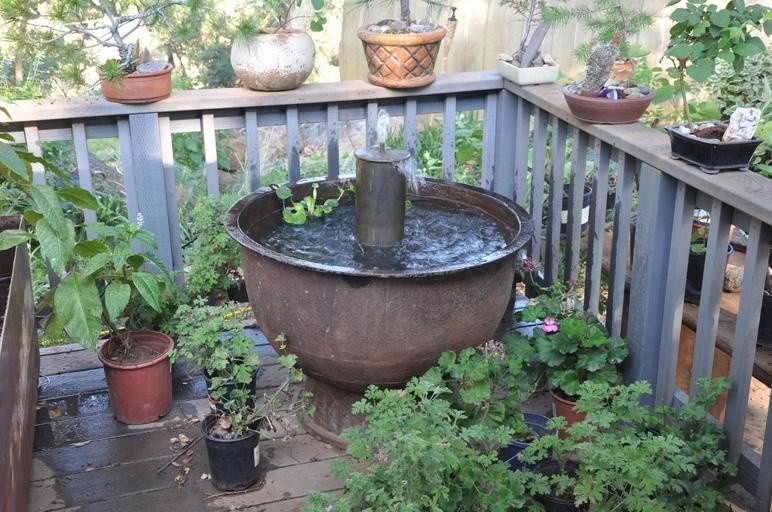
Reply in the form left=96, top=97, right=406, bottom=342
left=511, top=256, right=634, bottom=399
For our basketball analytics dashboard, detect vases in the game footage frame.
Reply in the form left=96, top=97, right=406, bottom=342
left=547, top=384, right=602, bottom=447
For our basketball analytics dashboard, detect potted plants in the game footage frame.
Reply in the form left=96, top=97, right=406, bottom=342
left=455, top=122, right=623, bottom=240
left=682, top=206, right=734, bottom=305
